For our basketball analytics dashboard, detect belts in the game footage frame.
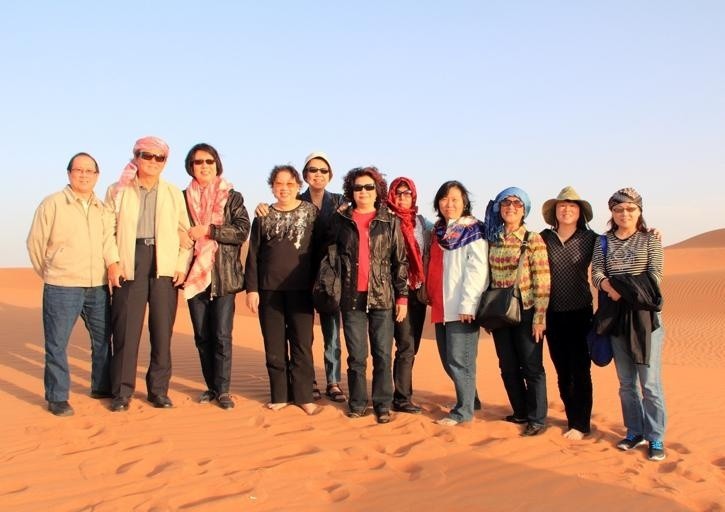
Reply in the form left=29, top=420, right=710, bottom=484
left=136, top=237, right=156, bottom=247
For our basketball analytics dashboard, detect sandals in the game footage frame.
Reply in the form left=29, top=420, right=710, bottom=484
left=308, top=378, right=322, bottom=401
left=325, top=381, right=346, bottom=403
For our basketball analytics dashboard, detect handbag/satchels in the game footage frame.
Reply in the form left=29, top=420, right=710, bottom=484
left=475, top=284, right=524, bottom=334
left=311, top=244, right=344, bottom=317
left=586, top=302, right=614, bottom=368
left=417, top=213, right=433, bottom=306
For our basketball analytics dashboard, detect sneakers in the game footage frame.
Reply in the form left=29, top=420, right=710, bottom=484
left=348, top=402, right=368, bottom=417
left=216, top=389, right=235, bottom=410
left=91, top=385, right=120, bottom=399
left=617, top=430, right=647, bottom=451
left=47, top=399, right=74, bottom=417
left=648, top=432, right=666, bottom=461
left=393, top=400, right=422, bottom=411
left=378, top=410, right=390, bottom=423
left=506, top=415, right=546, bottom=438
left=199, top=385, right=219, bottom=404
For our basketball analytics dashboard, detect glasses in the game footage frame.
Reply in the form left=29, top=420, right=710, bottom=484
left=309, top=166, right=330, bottom=174
left=501, top=199, right=525, bottom=209
left=393, top=189, right=413, bottom=198
left=194, top=159, right=217, bottom=165
left=140, top=151, right=167, bottom=163
left=353, top=183, right=378, bottom=192
left=613, top=207, right=639, bottom=213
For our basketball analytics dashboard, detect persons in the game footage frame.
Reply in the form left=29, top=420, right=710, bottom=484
left=106, top=137, right=194, bottom=412
left=325, top=168, right=408, bottom=423
left=179, top=144, right=250, bottom=410
left=591, top=188, right=667, bottom=461
left=425, top=180, right=490, bottom=427
left=246, top=165, right=323, bottom=415
left=539, top=186, right=663, bottom=441
left=255, top=154, right=348, bottom=402
left=386, top=177, right=435, bottom=412
left=483, top=187, right=552, bottom=437
left=26, top=152, right=113, bottom=417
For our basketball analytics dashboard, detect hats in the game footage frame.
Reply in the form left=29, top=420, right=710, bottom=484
left=133, top=136, right=170, bottom=161
left=493, top=186, right=531, bottom=223
left=607, top=187, right=643, bottom=212
left=542, top=186, right=594, bottom=226
left=302, top=152, right=334, bottom=185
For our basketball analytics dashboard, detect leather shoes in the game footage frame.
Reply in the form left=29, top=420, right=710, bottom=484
left=147, top=391, right=173, bottom=409
left=111, top=391, right=130, bottom=412
